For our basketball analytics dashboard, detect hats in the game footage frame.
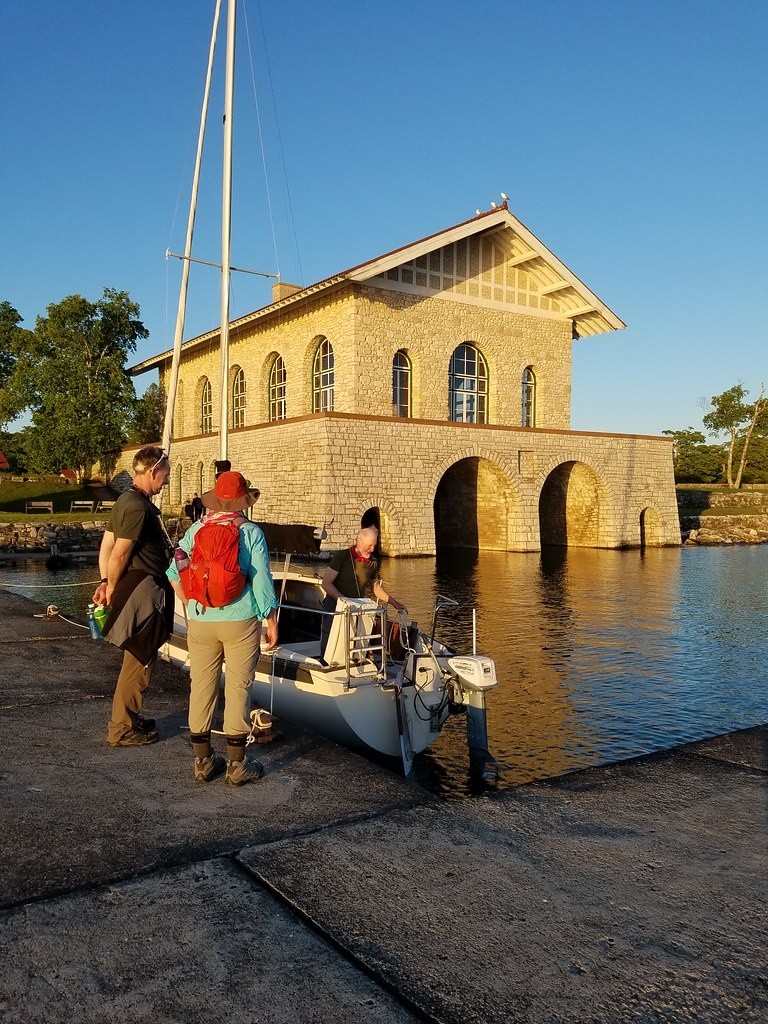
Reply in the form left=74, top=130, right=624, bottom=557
left=201, top=471, right=260, bottom=512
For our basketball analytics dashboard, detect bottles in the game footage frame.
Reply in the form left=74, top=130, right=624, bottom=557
left=175, top=548, right=189, bottom=571
left=93, top=605, right=108, bottom=632
left=86, top=604, right=105, bottom=639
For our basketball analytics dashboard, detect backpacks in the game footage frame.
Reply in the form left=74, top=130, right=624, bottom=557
left=177, top=516, right=249, bottom=607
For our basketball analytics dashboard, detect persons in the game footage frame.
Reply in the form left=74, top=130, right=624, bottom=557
left=92, top=447, right=171, bottom=747
left=185, top=500, right=194, bottom=521
left=166, top=472, right=280, bottom=786
left=192, top=493, right=204, bottom=521
left=320, top=528, right=409, bottom=666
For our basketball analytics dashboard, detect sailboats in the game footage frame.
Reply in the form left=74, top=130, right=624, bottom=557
left=152, top=0, right=503, bottom=789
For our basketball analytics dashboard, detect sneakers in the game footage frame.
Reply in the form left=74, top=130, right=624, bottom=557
left=107, top=727, right=159, bottom=747
left=130, top=712, right=157, bottom=734
left=224, top=754, right=264, bottom=787
left=194, top=750, right=225, bottom=783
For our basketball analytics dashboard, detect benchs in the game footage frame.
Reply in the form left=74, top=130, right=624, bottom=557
left=95, top=501, right=116, bottom=513
left=25, top=501, right=53, bottom=514
left=70, top=501, right=94, bottom=514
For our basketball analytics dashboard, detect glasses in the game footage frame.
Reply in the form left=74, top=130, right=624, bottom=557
left=150, top=453, right=168, bottom=472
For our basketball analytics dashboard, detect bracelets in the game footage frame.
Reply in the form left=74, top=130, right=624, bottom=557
left=101, top=578, right=108, bottom=583
